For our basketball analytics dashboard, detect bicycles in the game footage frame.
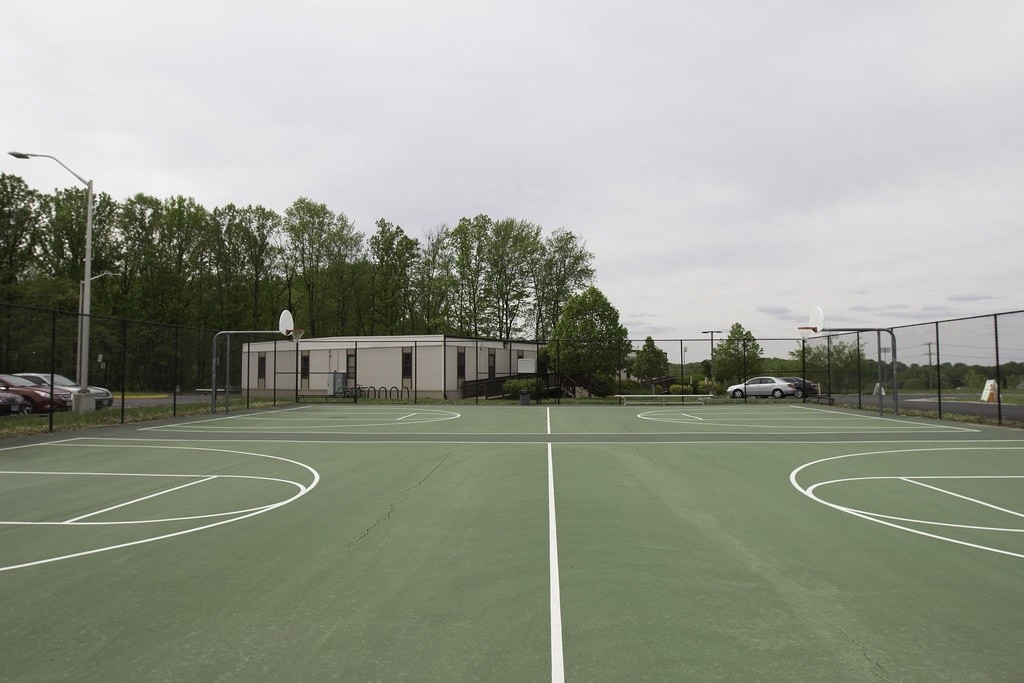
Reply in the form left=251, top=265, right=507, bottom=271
left=343, top=384, right=367, bottom=398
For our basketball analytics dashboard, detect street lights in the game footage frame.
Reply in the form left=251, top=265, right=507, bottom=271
left=9, top=149, right=96, bottom=390
left=76, top=271, right=125, bottom=383
left=701, top=330, right=722, bottom=386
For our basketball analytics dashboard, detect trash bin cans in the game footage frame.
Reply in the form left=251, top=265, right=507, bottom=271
left=520, top=390, right=531, bottom=405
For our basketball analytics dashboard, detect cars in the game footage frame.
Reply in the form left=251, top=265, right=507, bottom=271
left=755, top=377, right=819, bottom=399
left=726, top=376, right=797, bottom=399
left=11, top=373, right=114, bottom=409
left=0, top=374, right=73, bottom=415
left=0, top=383, right=27, bottom=418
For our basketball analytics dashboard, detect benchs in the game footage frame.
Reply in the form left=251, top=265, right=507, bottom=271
left=614, top=394, right=714, bottom=406
